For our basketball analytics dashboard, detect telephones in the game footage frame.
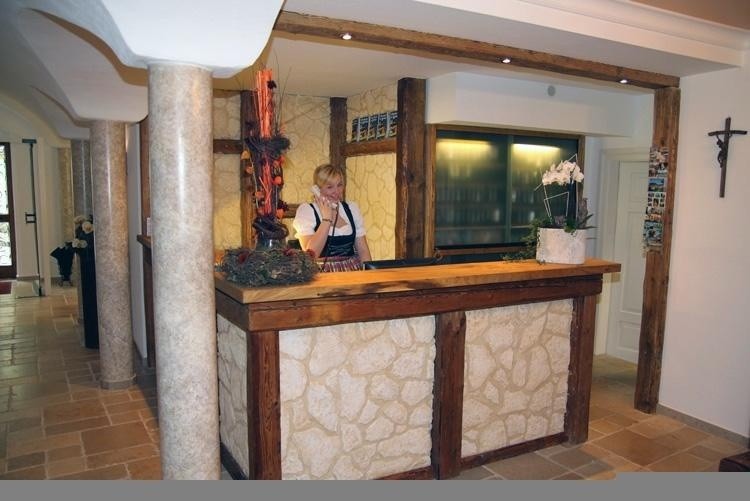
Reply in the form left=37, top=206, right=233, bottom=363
left=311, top=185, right=338, bottom=210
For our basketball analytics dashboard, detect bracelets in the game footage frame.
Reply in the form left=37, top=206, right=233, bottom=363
left=322, top=218, right=332, bottom=222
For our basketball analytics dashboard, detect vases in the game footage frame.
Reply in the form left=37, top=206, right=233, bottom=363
left=80, top=258, right=99, bottom=350
left=535, top=227, right=586, bottom=265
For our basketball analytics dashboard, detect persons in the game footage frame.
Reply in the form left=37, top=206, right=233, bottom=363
left=294, top=164, right=372, bottom=272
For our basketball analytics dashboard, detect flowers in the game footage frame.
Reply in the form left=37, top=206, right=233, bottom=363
left=71, top=216, right=94, bottom=258
left=542, top=160, right=585, bottom=232
left=221, top=67, right=315, bottom=287
